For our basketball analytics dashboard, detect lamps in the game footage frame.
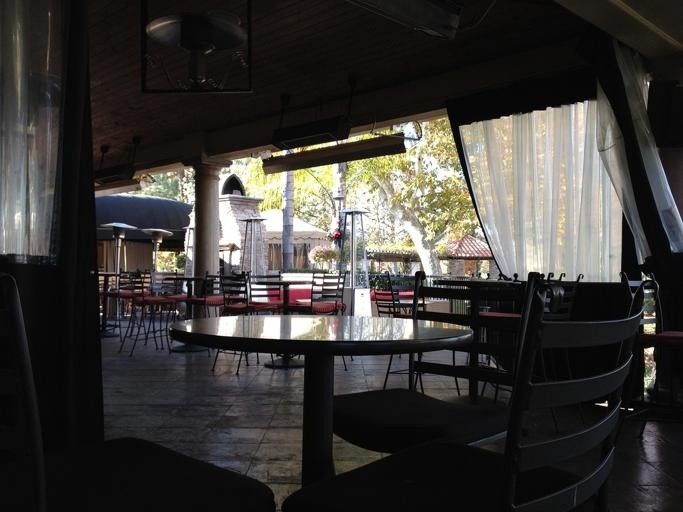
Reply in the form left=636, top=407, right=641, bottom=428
left=263, top=129, right=408, bottom=177
left=221, top=170, right=247, bottom=197
left=96, top=176, right=145, bottom=198
left=350, top=0, right=464, bottom=43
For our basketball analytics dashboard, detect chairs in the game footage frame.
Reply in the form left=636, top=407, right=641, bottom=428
left=0, top=271, right=682, bottom=511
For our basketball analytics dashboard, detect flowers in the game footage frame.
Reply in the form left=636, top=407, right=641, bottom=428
left=327, top=227, right=341, bottom=244
